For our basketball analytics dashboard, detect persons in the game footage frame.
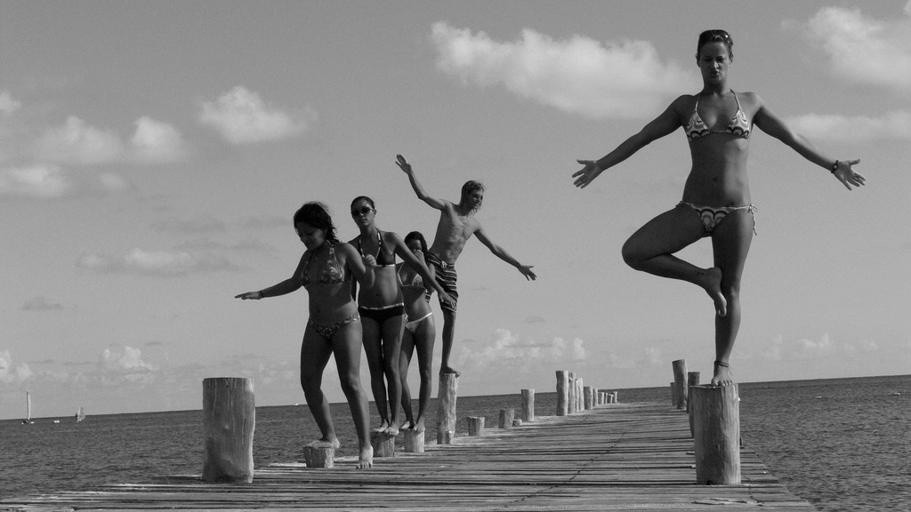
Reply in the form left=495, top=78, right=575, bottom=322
left=394, top=153, right=538, bottom=375
left=396, top=229, right=436, bottom=434
left=347, top=194, right=458, bottom=435
left=233, top=202, right=378, bottom=470
left=572, top=30, right=867, bottom=387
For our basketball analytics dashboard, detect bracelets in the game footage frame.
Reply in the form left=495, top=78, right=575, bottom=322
left=259, top=289, right=265, bottom=298
left=831, top=158, right=839, bottom=173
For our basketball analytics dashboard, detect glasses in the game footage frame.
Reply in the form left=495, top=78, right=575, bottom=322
left=351, top=207, right=376, bottom=217
left=700, top=30, right=729, bottom=41
